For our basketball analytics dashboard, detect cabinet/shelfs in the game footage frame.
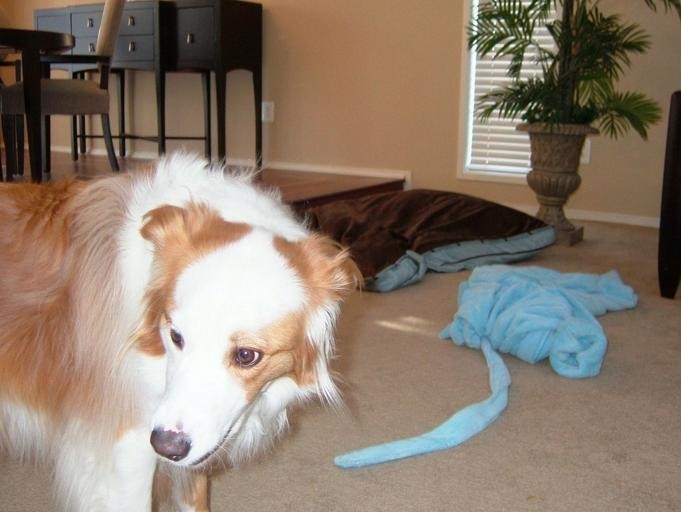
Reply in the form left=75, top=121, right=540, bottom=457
left=29, top=0, right=265, bottom=184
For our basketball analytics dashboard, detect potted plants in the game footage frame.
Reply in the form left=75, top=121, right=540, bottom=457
left=464, top=0, right=681, bottom=250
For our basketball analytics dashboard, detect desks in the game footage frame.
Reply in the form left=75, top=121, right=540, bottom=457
left=0, top=28, right=75, bottom=185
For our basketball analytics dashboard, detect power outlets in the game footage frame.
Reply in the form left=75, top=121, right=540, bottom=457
left=262, top=101, right=275, bottom=122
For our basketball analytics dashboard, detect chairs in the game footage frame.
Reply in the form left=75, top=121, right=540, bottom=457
left=1, top=1, right=125, bottom=180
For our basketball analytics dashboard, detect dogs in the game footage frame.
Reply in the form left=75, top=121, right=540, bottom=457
left=1, top=146, right=368, bottom=512
